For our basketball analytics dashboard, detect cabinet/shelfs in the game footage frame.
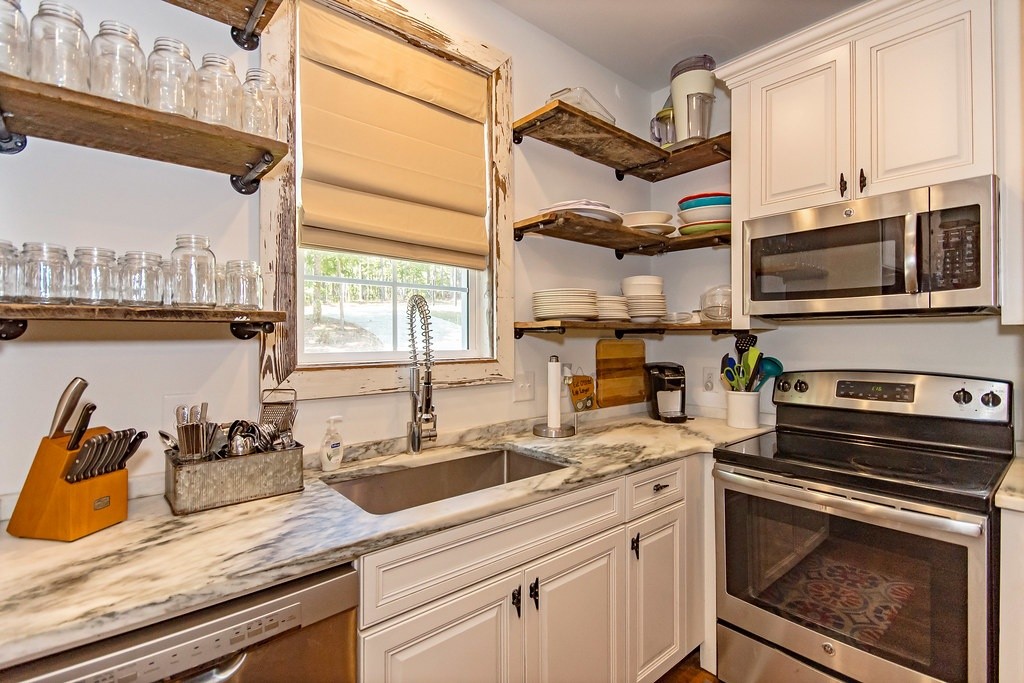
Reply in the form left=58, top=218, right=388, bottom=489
left=514, top=0, right=1024, bottom=340
left=0, top=0, right=289, bottom=340
left=350, top=453, right=715, bottom=683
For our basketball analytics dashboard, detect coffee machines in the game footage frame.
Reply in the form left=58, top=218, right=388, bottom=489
left=643, top=362, right=687, bottom=422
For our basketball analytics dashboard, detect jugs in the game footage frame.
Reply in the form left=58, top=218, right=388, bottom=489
left=650, top=54, right=716, bottom=152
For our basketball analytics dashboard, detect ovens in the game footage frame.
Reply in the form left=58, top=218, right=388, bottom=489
left=712, top=458, right=999, bottom=683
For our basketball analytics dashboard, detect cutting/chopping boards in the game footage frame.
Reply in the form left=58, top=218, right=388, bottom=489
left=596, top=339, right=646, bottom=407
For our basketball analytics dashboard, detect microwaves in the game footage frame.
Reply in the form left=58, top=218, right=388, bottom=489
left=742, top=175, right=1000, bottom=323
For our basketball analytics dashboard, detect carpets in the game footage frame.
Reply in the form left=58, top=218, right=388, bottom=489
left=763, top=552, right=917, bottom=642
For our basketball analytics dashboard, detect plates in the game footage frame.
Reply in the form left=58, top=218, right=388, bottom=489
left=531, top=287, right=668, bottom=321
left=540, top=190, right=731, bottom=235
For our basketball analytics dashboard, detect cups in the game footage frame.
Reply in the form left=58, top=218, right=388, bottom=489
left=726, top=391, right=761, bottom=428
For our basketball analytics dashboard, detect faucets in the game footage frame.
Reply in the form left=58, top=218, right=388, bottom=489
left=406, top=294, right=438, bottom=456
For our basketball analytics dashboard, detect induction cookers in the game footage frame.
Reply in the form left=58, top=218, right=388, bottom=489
left=713, top=369, right=1013, bottom=511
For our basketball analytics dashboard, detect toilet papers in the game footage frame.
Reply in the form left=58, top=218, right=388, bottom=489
left=548, top=361, right=561, bottom=428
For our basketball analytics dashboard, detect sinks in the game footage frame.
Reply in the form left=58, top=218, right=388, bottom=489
left=317, top=443, right=582, bottom=516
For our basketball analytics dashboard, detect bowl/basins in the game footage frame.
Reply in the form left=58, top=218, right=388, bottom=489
left=699, top=286, right=732, bottom=328
left=621, top=275, right=663, bottom=294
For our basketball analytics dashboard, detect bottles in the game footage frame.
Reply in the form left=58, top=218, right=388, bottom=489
left=561, top=363, right=575, bottom=413
left=1, top=0, right=281, bottom=141
left=0, top=235, right=264, bottom=309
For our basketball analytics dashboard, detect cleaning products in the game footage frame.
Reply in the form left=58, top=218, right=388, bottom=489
left=319, top=415, right=344, bottom=472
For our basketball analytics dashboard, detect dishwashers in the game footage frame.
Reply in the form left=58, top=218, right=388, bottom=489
left=0, top=567, right=357, bottom=683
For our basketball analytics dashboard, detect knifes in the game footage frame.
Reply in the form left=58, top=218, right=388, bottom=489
left=49, top=377, right=149, bottom=483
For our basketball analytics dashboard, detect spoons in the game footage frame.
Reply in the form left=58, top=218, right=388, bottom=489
left=158, top=430, right=179, bottom=451
